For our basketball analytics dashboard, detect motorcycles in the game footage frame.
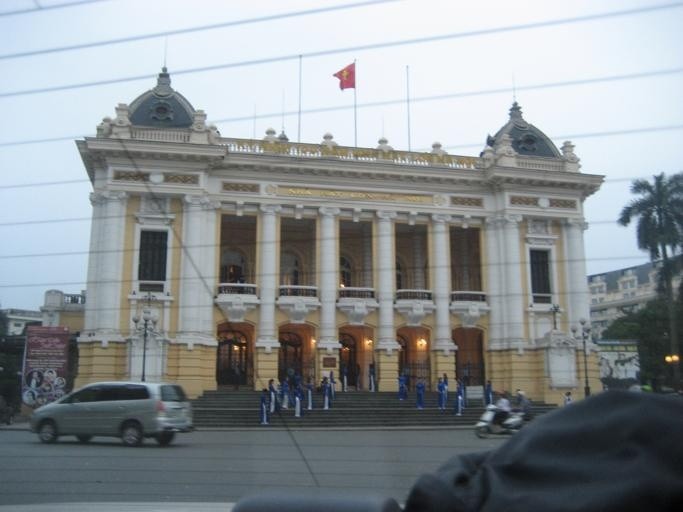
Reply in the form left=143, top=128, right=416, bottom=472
left=471, top=399, right=526, bottom=440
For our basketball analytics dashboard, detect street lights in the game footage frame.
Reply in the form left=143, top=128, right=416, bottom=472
left=662, top=353, right=681, bottom=394
left=569, top=317, right=593, bottom=399
left=129, top=307, right=161, bottom=381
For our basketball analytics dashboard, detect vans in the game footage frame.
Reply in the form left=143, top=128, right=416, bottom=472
left=29, top=380, right=195, bottom=445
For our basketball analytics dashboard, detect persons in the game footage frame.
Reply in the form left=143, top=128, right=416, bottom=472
left=237, top=276, right=248, bottom=293
left=598, top=353, right=640, bottom=379
left=494, top=392, right=510, bottom=423
left=564, top=392, right=573, bottom=406
left=260, top=363, right=493, bottom=425
left=26, top=370, right=64, bottom=407
left=518, top=389, right=533, bottom=416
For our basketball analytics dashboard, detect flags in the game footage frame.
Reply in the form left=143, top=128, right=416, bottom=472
left=334, top=63, right=355, bottom=90
